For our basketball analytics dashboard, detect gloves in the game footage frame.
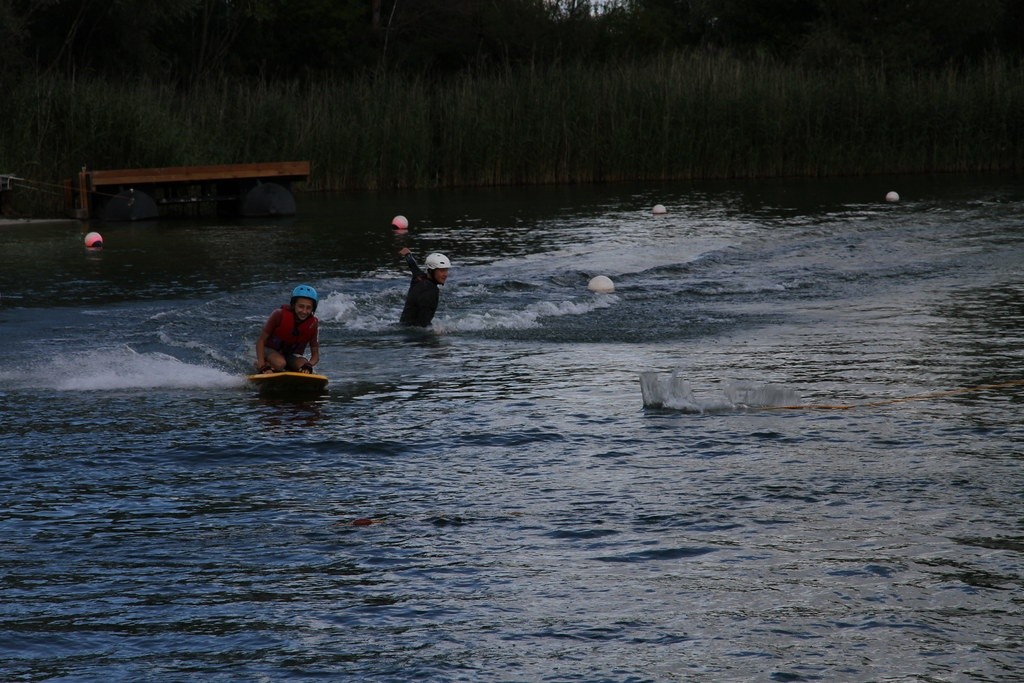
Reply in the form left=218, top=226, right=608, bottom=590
left=399, top=248, right=411, bottom=258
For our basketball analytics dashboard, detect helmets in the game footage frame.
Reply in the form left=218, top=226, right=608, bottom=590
left=290, top=285, right=318, bottom=313
left=425, top=253, right=451, bottom=270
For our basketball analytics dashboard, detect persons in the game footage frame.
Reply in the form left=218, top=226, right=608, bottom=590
left=256, top=285, right=320, bottom=374
left=399, top=247, right=453, bottom=327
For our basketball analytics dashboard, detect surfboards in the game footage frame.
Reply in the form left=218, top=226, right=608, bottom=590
left=247, top=372, right=329, bottom=386
